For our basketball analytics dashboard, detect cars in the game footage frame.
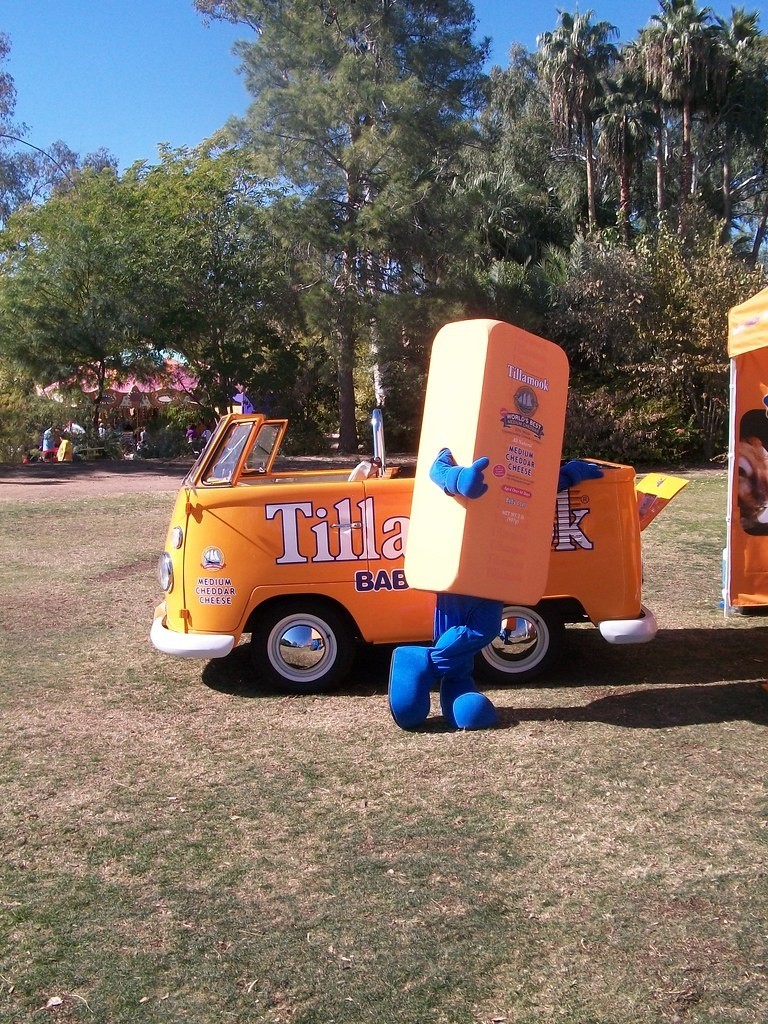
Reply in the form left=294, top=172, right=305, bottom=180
left=149, top=411, right=690, bottom=693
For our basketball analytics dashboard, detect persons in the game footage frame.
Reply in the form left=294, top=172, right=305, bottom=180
left=17, top=419, right=212, bottom=464
left=383, top=320, right=604, bottom=732
